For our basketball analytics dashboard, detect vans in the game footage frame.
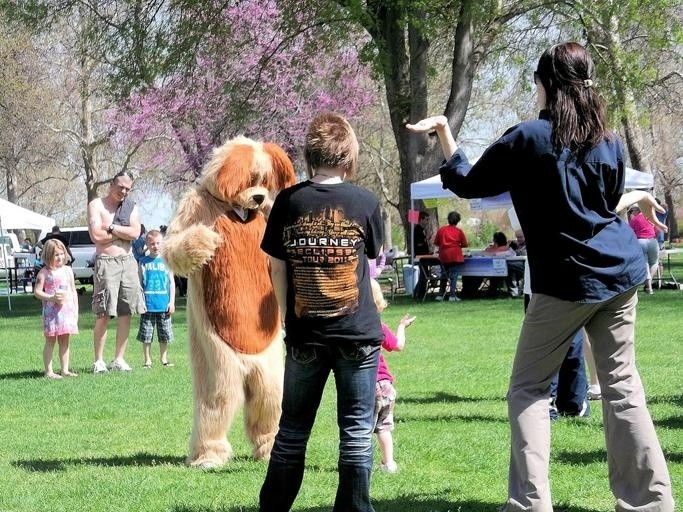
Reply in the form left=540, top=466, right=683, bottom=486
left=0, top=233, right=19, bottom=278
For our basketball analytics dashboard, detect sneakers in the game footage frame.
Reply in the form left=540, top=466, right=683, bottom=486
left=377, top=457, right=398, bottom=474
left=434, top=295, right=462, bottom=305
left=551, top=382, right=604, bottom=418
left=43, top=360, right=179, bottom=381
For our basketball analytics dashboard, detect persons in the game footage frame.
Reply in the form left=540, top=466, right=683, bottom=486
left=259, top=113, right=387, bottom=512
left=87, top=172, right=148, bottom=375
left=21, top=226, right=78, bottom=378
left=406, top=42, right=676, bottom=512
left=581, top=326, right=602, bottom=400
left=630, top=198, right=669, bottom=295
left=132, top=224, right=176, bottom=368
left=369, top=278, right=416, bottom=472
left=366, top=244, right=388, bottom=278
left=414, top=211, right=527, bottom=302
left=548, top=328, right=590, bottom=420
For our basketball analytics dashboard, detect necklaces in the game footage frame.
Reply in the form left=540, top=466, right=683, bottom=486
left=315, top=173, right=342, bottom=181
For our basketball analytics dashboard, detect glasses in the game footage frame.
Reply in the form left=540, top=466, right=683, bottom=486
left=532, top=70, right=542, bottom=85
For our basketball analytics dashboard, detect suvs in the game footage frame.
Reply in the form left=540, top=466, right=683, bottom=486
left=32, top=227, right=97, bottom=285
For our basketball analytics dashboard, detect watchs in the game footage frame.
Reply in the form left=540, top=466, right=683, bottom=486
left=107, top=224, right=114, bottom=233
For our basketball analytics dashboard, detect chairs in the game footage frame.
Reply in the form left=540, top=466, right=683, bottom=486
left=416, top=255, right=455, bottom=304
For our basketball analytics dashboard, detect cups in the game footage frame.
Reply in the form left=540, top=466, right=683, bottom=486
left=55, top=290, right=66, bottom=305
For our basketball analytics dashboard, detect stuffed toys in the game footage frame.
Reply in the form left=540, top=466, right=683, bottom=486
left=163, top=135, right=296, bottom=472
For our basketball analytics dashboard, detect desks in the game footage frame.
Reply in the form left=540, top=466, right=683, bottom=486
left=656, top=244, right=683, bottom=288
left=414, top=254, right=525, bottom=297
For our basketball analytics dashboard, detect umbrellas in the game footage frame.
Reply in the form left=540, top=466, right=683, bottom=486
left=2, top=197, right=56, bottom=310
